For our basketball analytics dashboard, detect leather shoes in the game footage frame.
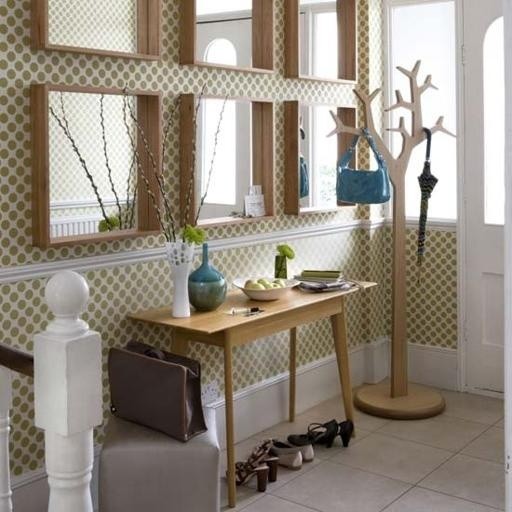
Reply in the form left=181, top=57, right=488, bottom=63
left=261, top=438, right=315, bottom=471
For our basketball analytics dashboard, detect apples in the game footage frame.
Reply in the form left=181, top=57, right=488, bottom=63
left=245, top=278, right=285, bottom=290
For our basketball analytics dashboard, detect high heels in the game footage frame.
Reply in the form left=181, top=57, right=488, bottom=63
left=225, top=449, right=278, bottom=494
left=287, top=420, right=354, bottom=449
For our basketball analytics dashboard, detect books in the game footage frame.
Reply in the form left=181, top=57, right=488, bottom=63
left=294, top=268, right=345, bottom=283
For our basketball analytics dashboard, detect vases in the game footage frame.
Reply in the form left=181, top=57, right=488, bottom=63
left=273, top=255, right=288, bottom=281
left=160, top=235, right=196, bottom=319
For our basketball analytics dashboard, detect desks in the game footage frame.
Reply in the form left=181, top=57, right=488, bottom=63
left=120, top=272, right=380, bottom=507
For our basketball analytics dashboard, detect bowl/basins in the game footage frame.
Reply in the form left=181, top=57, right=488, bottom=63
left=233, top=277, right=301, bottom=301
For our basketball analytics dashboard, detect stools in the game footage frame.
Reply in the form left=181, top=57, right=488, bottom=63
left=91, top=405, right=225, bottom=511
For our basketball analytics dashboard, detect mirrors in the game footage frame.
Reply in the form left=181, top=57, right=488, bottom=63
left=284, top=99, right=360, bottom=216
left=283, top=0, right=359, bottom=86
left=177, top=92, right=277, bottom=230
left=30, top=81, right=166, bottom=250
left=29, top=0, right=163, bottom=61
left=179, top=0, right=276, bottom=74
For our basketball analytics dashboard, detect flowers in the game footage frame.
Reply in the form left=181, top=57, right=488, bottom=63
left=274, top=244, right=296, bottom=279
left=97, top=214, right=122, bottom=234
left=179, top=221, right=208, bottom=248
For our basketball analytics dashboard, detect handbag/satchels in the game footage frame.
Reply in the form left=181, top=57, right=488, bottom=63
left=108, top=340, right=208, bottom=444
left=334, top=128, right=392, bottom=206
